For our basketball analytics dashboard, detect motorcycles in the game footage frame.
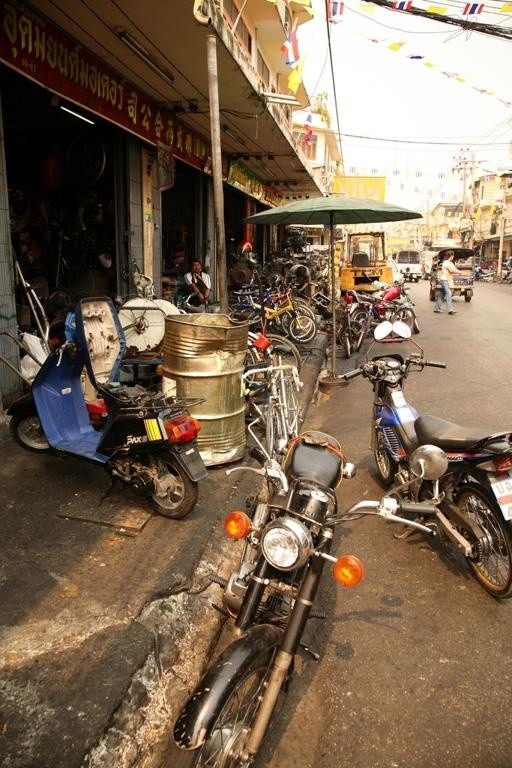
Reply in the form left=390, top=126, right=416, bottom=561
left=337, top=321, right=512, bottom=599
left=8, top=297, right=209, bottom=518
left=475, top=265, right=496, bottom=282
left=346, top=278, right=420, bottom=337
left=430, top=248, right=474, bottom=302
left=174, top=432, right=448, bottom=768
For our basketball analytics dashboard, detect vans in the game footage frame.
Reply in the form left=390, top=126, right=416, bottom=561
left=455, top=256, right=480, bottom=269
left=392, top=251, right=422, bottom=282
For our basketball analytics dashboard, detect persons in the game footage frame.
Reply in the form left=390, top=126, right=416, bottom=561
left=505, top=256, right=512, bottom=271
left=433, top=250, right=462, bottom=315
left=289, top=262, right=311, bottom=298
left=181, top=259, right=212, bottom=306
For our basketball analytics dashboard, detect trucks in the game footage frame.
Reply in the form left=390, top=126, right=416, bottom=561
left=421, top=239, right=457, bottom=279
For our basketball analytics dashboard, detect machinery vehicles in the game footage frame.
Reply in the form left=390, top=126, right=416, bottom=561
left=340, top=232, right=394, bottom=296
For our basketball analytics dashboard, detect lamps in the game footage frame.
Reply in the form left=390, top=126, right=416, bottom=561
left=259, top=93, right=302, bottom=116
left=223, top=125, right=245, bottom=146
left=118, top=32, right=175, bottom=84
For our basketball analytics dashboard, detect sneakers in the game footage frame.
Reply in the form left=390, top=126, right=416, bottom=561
left=449, top=311, right=457, bottom=315
left=434, top=310, right=443, bottom=313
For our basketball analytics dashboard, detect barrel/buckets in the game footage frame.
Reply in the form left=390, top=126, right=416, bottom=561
left=162, top=313, right=248, bottom=468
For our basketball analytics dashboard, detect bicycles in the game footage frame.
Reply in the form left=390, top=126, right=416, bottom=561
left=356, top=291, right=420, bottom=358
left=230, top=270, right=317, bottom=461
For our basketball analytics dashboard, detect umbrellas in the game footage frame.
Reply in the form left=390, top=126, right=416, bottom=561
left=245, top=195, right=423, bottom=375
